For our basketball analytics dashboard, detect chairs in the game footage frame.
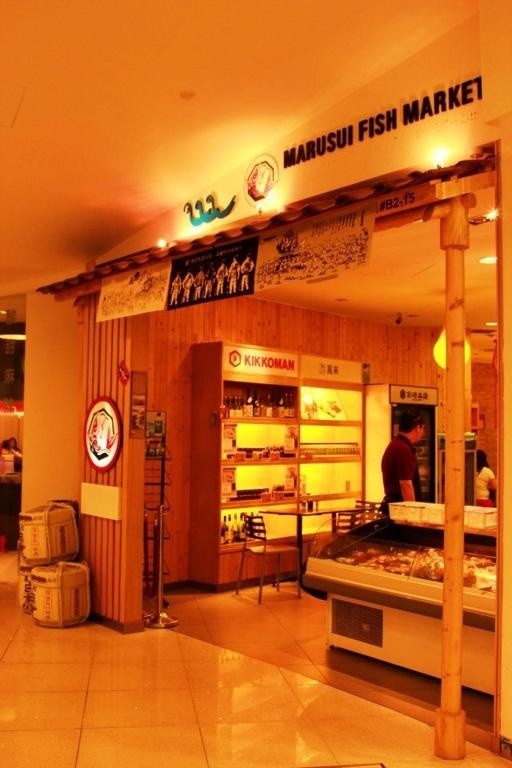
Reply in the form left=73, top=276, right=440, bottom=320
left=330, top=510, right=366, bottom=535
left=236, top=513, right=302, bottom=605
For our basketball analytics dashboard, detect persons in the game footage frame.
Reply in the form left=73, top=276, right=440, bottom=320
left=6, top=437, right=23, bottom=471
left=474, top=449, right=498, bottom=507
left=170, top=253, right=255, bottom=307
left=381, top=407, right=427, bottom=513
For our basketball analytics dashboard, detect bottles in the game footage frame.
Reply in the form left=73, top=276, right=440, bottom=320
left=344, top=478, right=351, bottom=492
left=222, top=390, right=295, bottom=418
left=230, top=485, right=285, bottom=502
left=299, top=441, right=360, bottom=456
left=153, top=411, right=164, bottom=438
left=144, top=439, right=165, bottom=459
left=301, top=498, right=320, bottom=510
left=221, top=512, right=265, bottom=544
left=395, top=412, right=430, bottom=491
left=236, top=441, right=285, bottom=458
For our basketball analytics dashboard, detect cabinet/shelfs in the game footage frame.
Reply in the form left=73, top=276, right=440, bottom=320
left=303, top=518, right=497, bottom=697
left=190, top=341, right=367, bottom=584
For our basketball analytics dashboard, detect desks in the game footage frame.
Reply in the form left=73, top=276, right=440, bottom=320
left=259, top=509, right=344, bottom=583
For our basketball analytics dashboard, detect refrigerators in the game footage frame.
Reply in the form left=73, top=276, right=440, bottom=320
left=436, top=432, right=477, bottom=508
left=365, top=384, right=439, bottom=506
